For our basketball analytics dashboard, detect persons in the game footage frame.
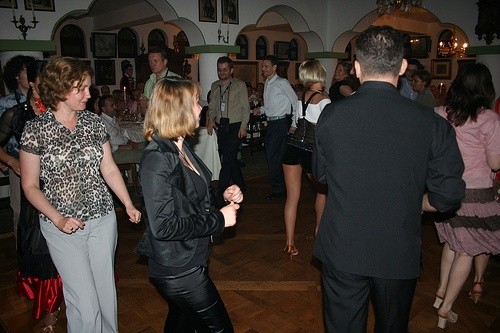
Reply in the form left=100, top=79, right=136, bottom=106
left=254, top=56, right=299, bottom=203
left=0, top=51, right=264, bottom=333
left=272, top=25, right=500, bottom=333
left=21, top=54, right=144, bottom=333
left=205, top=56, right=251, bottom=195
left=420, top=60, right=499, bottom=328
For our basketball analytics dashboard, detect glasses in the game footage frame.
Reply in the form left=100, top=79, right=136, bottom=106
left=414, top=80, right=422, bottom=83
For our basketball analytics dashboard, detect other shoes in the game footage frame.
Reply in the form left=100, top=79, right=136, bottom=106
left=40, top=306, right=61, bottom=333
left=265, top=193, right=280, bottom=201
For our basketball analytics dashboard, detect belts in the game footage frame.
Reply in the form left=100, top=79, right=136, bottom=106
left=267, top=116, right=286, bottom=121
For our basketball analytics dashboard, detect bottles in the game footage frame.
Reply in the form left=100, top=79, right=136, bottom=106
left=123, top=100, right=129, bottom=121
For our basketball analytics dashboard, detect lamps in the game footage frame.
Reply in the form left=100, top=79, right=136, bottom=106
left=436, top=22, right=469, bottom=59
left=11, top=1, right=40, bottom=40
left=376, top=0, right=424, bottom=16
left=217, top=17, right=230, bottom=44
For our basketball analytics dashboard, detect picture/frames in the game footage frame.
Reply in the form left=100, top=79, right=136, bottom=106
left=93, top=60, right=117, bottom=86
left=198, top=0, right=217, bottom=23
left=0, top=0, right=19, bottom=9
left=91, top=32, right=118, bottom=59
left=23, top=0, right=56, bottom=13
left=220, top=0, right=240, bottom=25
left=429, top=58, right=452, bottom=81
left=230, top=59, right=260, bottom=90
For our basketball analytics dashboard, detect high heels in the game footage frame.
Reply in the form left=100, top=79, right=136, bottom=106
left=438, top=310, right=459, bottom=329
left=286, top=245, right=299, bottom=260
left=433, top=295, right=443, bottom=309
left=469, top=282, right=485, bottom=303
